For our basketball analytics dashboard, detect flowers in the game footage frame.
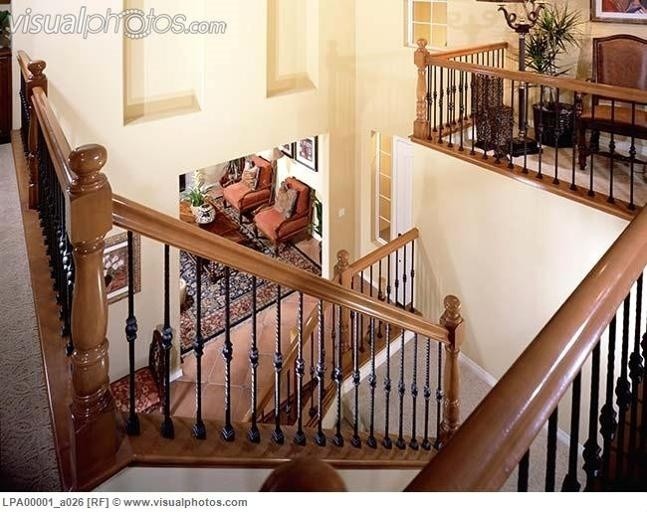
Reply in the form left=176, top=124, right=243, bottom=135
left=180, top=166, right=213, bottom=206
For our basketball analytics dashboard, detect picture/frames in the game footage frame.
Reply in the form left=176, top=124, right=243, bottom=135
left=101, top=229, right=142, bottom=306
left=589, top=0, right=647, bottom=26
left=294, top=135, right=318, bottom=173
left=279, top=141, right=294, bottom=158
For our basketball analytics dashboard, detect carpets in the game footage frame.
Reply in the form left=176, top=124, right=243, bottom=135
left=178, top=188, right=321, bottom=355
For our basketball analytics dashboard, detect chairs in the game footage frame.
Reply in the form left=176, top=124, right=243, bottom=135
left=252, top=176, right=316, bottom=259
left=572, top=33, right=647, bottom=186
left=108, top=329, right=166, bottom=416
left=222, top=154, right=278, bottom=226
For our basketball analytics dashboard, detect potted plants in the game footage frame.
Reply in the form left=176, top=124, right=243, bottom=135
left=306, top=194, right=322, bottom=267
left=505, top=0, right=590, bottom=150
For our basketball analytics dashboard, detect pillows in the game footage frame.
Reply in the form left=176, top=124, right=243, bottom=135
left=240, top=161, right=261, bottom=191
left=271, top=181, right=300, bottom=219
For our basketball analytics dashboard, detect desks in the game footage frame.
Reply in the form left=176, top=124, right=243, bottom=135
left=179, top=200, right=253, bottom=285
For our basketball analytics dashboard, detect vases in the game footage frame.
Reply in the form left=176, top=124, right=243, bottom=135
left=189, top=202, right=206, bottom=216
